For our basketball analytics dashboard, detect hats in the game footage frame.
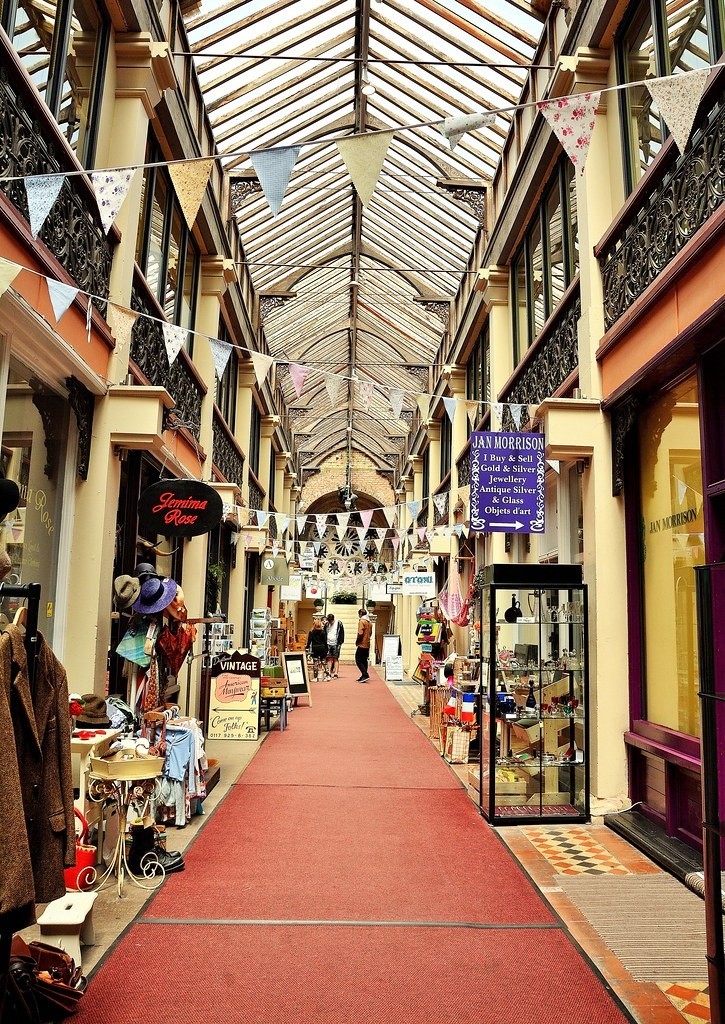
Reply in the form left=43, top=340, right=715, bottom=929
left=131, top=562, right=167, bottom=580
left=112, top=575, right=141, bottom=610
left=165, top=584, right=187, bottom=622
left=75, top=694, right=109, bottom=724
left=130, top=577, right=177, bottom=614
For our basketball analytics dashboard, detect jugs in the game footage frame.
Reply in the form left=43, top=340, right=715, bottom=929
left=528, top=591, right=546, bottom=622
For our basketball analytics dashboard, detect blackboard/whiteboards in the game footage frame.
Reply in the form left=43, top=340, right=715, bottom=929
left=281, top=650, right=311, bottom=697
left=386, top=656, right=403, bottom=680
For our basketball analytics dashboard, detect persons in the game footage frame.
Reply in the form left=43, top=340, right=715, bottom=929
left=305, top=614, right=344, bottom=682
left=355, top=609, right=372, bottom=682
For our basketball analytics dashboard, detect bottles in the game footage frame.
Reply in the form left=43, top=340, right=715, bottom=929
left=547, top=606, right=551, bottom=622
left=551, top=606, right=557, bottom=621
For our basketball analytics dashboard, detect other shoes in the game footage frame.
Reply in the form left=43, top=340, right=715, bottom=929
left=325, top=675, right=330, bottom=681
left=310, top=677, right=317, bottom=682
left=321, top=675, right=331, bottom=682
left=333, top=673, right=337, bottom=680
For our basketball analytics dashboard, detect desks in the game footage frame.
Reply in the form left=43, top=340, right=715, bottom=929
left=450, top=684, right=512, bottom=762
left=76, top=771, right=167, bottom=900
left=260, top=695, right=288, bottom=734
left=68, top=728, right=123, bottom=844
left=427, top=685, right=450, bottom=740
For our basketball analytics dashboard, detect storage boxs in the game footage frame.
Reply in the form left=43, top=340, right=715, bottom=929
left=263, top=665, right=282, bottom=678
left=89, top=749, right=166, bottom=776
left=261, top=688, right=285, bottom=698
left=468, top=766, right=528, bottom=807
left=261, top=676, right=270, bottom=686
left=268, top=678, right=287, bottom=688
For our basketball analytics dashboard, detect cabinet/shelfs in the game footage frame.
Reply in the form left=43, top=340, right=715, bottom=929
left=480, top=564, right=591, bottom=827
left=250, top=607, right=271, bottom=670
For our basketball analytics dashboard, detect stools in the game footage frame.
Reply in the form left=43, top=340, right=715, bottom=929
left=37, top=892, right=98, bottom=969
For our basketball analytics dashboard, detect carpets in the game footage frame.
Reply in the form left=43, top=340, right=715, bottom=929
left=556, top=872, right=725, bottom=983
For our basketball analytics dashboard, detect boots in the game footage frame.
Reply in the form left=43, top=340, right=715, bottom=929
left=127, top=817, right=184, bottom=876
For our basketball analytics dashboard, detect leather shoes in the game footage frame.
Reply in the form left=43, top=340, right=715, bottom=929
left=355, top=675, right=369, bottom=683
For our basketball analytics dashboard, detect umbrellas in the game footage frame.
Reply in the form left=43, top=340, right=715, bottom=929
left=156, top=616, right=196, bottom=685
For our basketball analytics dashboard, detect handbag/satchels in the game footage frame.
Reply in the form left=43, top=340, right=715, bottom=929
left=60, top=807, right=97, bottom=892
left=22, top=941, right=88, bottom=1012
left=443, top=726, right=469, bottom=764
